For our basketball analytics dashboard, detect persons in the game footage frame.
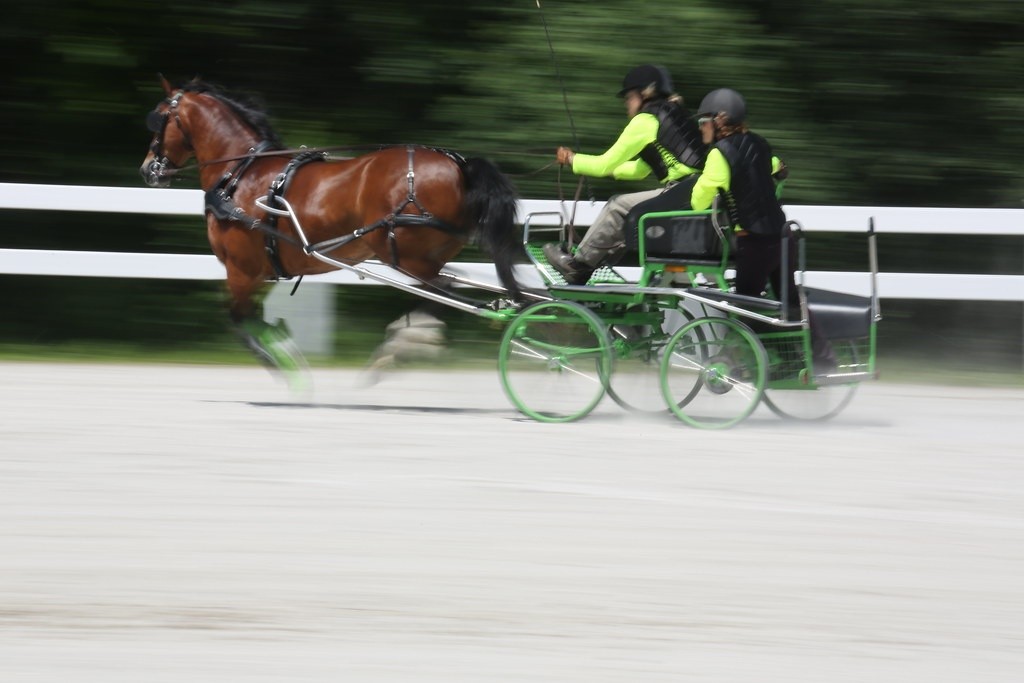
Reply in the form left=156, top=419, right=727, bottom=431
left=690, top=88, right=836, bottom=374
left=544, top=64, right=703, bottom=287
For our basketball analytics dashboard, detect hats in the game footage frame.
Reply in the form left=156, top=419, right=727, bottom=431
left=616, top=66, right=667, bottom=99
left=688, top=89, right=745, bottom=121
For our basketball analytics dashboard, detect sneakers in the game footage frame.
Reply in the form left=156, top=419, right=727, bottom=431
left=542, top=244, right=592, bottom=286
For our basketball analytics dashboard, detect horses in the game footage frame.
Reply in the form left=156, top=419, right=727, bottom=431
left=138, top=74, right=529, bottom=393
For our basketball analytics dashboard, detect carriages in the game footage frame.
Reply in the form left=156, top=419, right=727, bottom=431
left=140, top=70, right=882, bottom=432
